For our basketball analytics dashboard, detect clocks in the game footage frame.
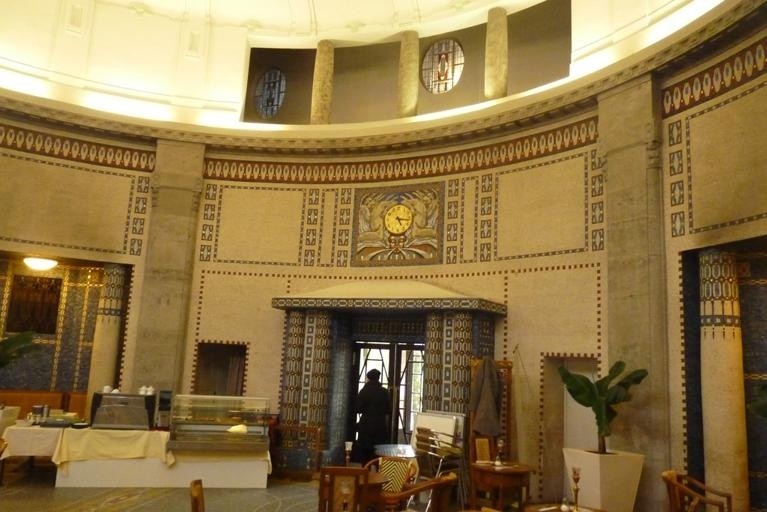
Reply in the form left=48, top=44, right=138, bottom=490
left=383, top=204, right=413, bottom=236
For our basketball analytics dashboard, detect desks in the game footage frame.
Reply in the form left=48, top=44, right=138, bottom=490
left=470, top=461, right=530, bottom=512
left=0, top=423, right=274, bottom=489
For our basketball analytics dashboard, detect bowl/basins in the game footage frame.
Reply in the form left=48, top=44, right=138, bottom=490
left=14, top=419, right=36, bottom=427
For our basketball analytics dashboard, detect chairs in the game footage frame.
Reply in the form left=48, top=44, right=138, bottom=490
left=661, top=469, right=732, bottom=512
left=318, top=427, right=467, bottom=512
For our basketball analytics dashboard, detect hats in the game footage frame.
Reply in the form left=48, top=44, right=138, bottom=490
left=367, top=369, right=380, bottom=381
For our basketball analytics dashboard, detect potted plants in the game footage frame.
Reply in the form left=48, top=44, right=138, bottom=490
left=559, top=360, right=648, bottom=512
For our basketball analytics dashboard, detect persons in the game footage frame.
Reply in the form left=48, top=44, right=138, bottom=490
left=352, top=369, right=401, bottom=467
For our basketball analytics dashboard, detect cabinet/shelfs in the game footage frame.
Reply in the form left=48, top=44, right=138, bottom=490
left=168, top=395, right=271, bottom=454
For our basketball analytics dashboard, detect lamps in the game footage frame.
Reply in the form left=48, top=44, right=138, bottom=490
left=23, top=258, right=58, bottom=270
